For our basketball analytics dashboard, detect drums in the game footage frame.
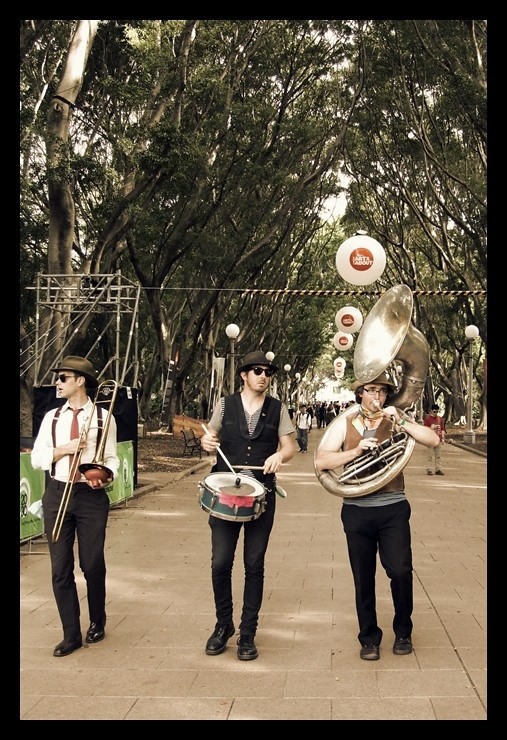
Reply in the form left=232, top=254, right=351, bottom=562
left=198, top=471, right=269, bottom=523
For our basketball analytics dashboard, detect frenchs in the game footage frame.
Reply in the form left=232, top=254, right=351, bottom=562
left=313, top=283, right=432, bottom=498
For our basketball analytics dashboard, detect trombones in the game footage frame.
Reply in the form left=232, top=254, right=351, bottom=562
left=51, top=376, right=120, bottom=544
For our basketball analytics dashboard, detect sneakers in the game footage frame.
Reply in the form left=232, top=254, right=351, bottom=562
left=393, top=634, right=412, bottom=655
left=360, top=644, right=380, bottom=660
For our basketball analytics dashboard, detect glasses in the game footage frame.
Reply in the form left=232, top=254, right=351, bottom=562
left=55, top=374, right=80, bottom=382
left=247, top=367, right=272, bottom=377
left=432, top=410, right=439, bottom=413
left=363, top=388, right=389, bottom=396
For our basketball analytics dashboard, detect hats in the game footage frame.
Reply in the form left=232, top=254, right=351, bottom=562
left=236, top=352, right=279, bottom=376
left=51, top=355, right=100, bottom=389
left=354, top=371, right=398, bottom=394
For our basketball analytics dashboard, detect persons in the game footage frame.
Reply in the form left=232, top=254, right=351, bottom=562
left=31, top=355, right=120, bottom=657
left=316, top=371, right=440, bottom=659
left=288, top=401, right=355, bottom=450
left=296, top=405, right=312, bottom=454
left=423, top=404, right=445, bottom=476
left=201, top=351, right=297, bottom=660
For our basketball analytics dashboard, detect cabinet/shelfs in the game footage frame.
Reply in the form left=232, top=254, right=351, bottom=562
left=34, top=268, right=140, bottom=388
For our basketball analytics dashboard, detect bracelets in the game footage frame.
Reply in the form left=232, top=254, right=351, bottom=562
left=442, top=438, right=445, bottom=439
left=401, top=419, right=405, bottom=425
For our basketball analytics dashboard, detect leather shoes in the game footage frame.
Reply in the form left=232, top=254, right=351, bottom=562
left=53, top=633, right=83, bottom=656
left=206, top=621, right=235, bottom=655
left=85, top=619, right=104, bottom=643
left=237, top=627, right=257, bottom=659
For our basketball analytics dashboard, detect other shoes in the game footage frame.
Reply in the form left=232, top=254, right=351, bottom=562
left=304, top=447, right=308, bottom=453
left=298, top=448, right=303, bottom=452
left=427, top=471, right=433, bottom=476
left=436, top=469, right=445, bottom=476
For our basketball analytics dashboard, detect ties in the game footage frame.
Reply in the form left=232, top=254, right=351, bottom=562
left=69, top=409, right=83, bottom=483
